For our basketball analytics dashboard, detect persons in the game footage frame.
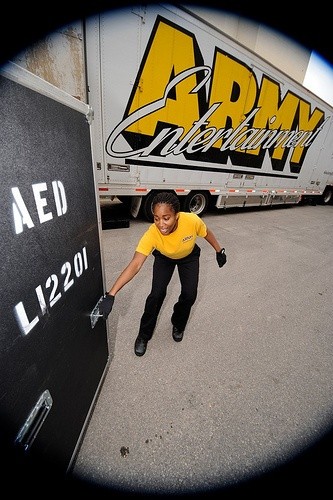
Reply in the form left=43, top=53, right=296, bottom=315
left=99, top=193, right=227, bottom=356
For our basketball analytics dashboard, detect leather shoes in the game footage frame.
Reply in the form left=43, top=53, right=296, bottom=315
left=173, top=325, right=183, bottom=341
left=135, top=336, right=148, bottom=355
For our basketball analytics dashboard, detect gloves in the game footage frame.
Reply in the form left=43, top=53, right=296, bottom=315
left=217, top=248, right=227, bottom=267
left=99, top=294, right=115, bottom=319
